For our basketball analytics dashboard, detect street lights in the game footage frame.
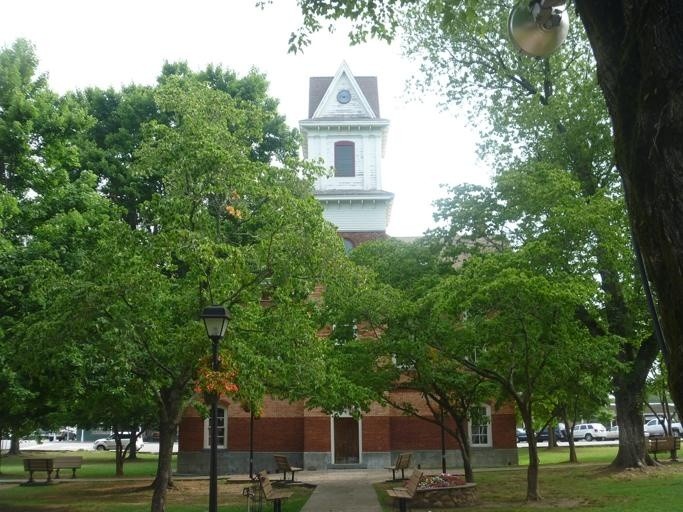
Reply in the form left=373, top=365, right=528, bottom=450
left=200, top=304, right=231, bottom=512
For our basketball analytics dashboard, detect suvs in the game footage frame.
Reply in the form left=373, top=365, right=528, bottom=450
left=93, top=432, right=144, bottom=451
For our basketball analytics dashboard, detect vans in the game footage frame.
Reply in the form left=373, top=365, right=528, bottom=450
left=573, top=423, right=607, bottom=441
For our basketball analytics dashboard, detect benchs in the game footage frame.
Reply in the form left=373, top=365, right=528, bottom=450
left=274, top=456, right=304, bottom=480
left=649, top=437, right=680, bottom=462
left=259, top=470, right=294, bottom=512
left=23, top=455, right=82, bottom=483
left=386, top=469, right=423, bottom=512
left=384, top=453, right=411, bottom=482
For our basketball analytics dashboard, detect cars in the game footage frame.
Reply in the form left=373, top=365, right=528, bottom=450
left=56, top=432, right=76, bottom=441
left=606, top=426, right=650, bottom=441
left=515, top=428, right=558, bottom=443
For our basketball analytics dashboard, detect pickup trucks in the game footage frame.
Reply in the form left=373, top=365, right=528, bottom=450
left=644, top=418, right=683, bottom=437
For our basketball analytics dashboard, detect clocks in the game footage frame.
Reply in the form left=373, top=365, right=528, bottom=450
left=337, top=90, right=351, bottom=104
